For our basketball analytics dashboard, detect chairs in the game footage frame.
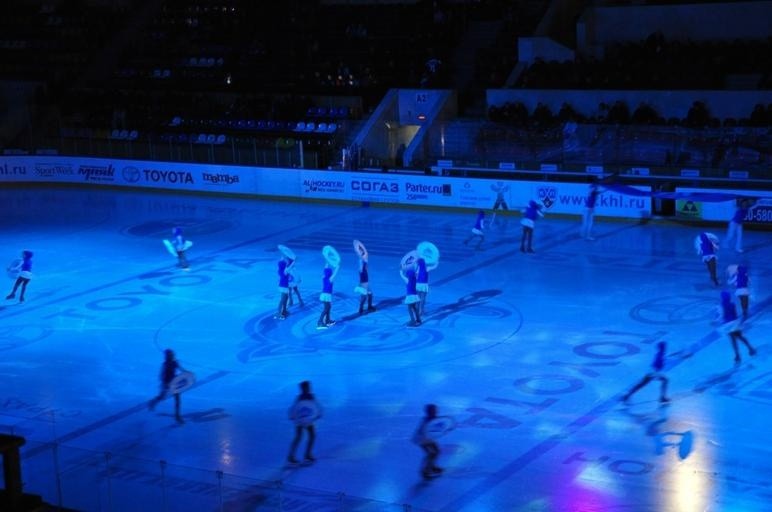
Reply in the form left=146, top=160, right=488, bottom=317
left=106, top=103, right=352, bottom=146
left=151, top=57, right=225, bottom=81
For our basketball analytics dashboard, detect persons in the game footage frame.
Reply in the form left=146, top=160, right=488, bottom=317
left=578, top=190, right=597, bottom=240
left=520, top=201, right=545, bottom=253
left=146, top=350, right=186, bottom=424
left=624, top=342, right=669, bottom=402
left=286, top=382, right=323, bottom=464
left=722, top=199, right=748, bottom=253
left=408, top=404, right=443, bottom=482
left=6, top=251, right=33, bottom=301
left=273, top=253, right=438, bottom=327
left=693, top=227, right=719, bottom=287
left=727, top=265, right=753, bottom=321
left=718, top=291, right=756, bottom=361
left=170, top=226, right=189, bottom=268
left=463, top=209, right=486, bottom=251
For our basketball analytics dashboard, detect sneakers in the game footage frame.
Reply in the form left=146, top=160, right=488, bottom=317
left=462, top=239, right=482, bottom=253
left=176, top=262, right=190, bottom=271
left=5, top=293, right=25, bottom=304
left=284, top=456, right=316, bottom=467
left=580, top=235, right=597, bottom=243
left=273, top=300, right=427, bottom=331
left=720, top=242, right=746, bottom=254
left=148, top=400, right=184, bottom=424
left=709, top=273, right=720, bottom=288
left=615, top=395, right=672, bottom=409
left=731, top=349, right=759, bottom=368
left=519, top=246, right=537, bottom=259
left=416, top=466, right=444, bottom=481
